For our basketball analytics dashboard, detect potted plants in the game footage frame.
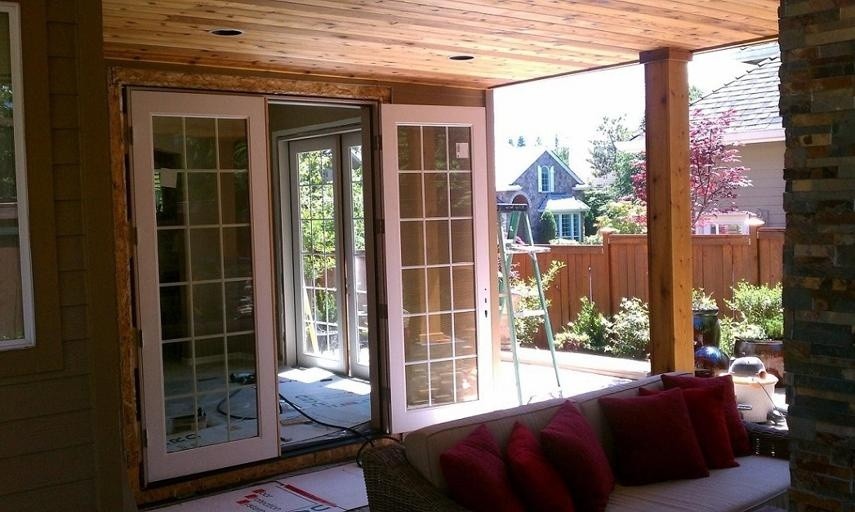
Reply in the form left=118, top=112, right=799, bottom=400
left=721, top=322, right=786, bottom=385
left=691, top=287, right=721, bottom=352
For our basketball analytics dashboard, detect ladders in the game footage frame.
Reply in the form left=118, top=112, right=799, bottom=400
left=496, top=202, right=563, bottom=404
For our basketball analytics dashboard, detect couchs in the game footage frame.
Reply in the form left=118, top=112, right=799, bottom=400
left=360, top=371, right=792, bottom=511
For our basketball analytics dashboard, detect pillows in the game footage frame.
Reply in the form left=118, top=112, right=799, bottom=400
left=539, top=398, right=615, bottom=510
left=662, top=374, right=753, bottom=457
left=639, top=384, right=739, bottom=468
left=504, top=419, right=577, bottom=512
left=438, top=422, right=526, bottom=512
left=599, top=386, right=709, bottom=487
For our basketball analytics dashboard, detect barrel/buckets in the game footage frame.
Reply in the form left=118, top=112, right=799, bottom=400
left=720, top=373, right=779, bottom=423
